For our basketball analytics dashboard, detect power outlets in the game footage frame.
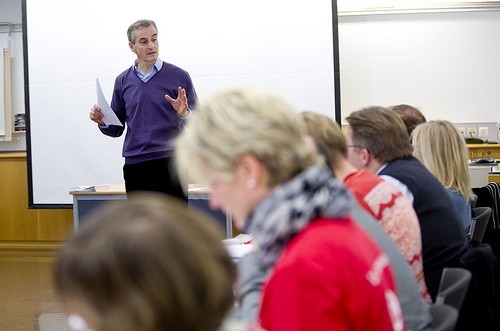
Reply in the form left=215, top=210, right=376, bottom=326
left=467, top=128, right=478, bottom=137
left=458, top=128, right=466, bottom=137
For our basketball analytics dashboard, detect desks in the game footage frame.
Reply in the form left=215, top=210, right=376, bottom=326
left=70, top=186, right=232, bottom=239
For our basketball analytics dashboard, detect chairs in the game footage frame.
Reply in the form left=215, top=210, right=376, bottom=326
left=469, top=206, right=492, bottom=242
left=427, top=303, right=459, bottom=331
left=436, top=267, right=472, bottom=311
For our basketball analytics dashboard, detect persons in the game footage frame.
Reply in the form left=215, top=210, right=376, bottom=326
left=175, top=83, right=427, bottom=331
left=345, top=107, right=468, bottom=302
left=299, top=109, right=433, bottom=303
left=391, top=104, right=426, bottom=133
left=89, top=19, right=200, bottom=207
left=410, top=120, right=478, bottom=234
left=49, top=189, right=238, bottom=331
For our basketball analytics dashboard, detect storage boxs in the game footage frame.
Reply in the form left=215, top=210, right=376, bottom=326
left=467, top=166, right=492, bottom=189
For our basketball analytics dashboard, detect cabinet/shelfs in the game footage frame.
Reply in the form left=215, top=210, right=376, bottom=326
left=466, top=142, right=500, bottom=184
